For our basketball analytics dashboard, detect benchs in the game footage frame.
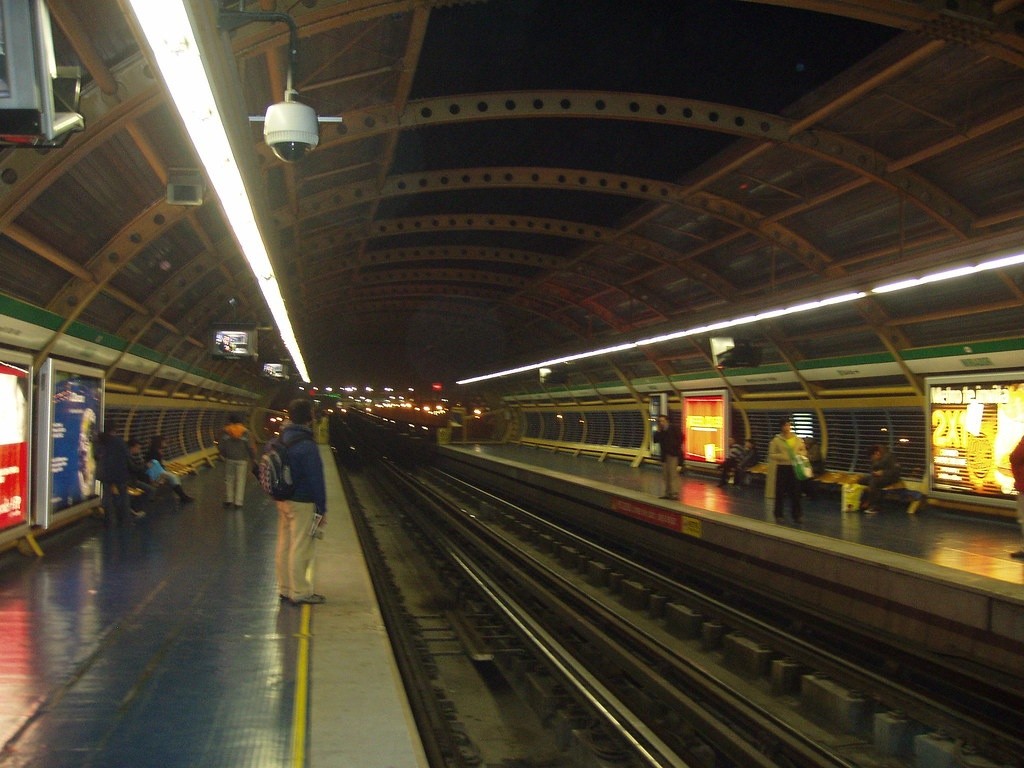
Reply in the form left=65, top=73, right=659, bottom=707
left=746, top=460, right=927, bottom=515
left=112, top=461, right=196, bottom=508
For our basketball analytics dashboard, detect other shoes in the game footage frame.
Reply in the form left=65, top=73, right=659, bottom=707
left=278, top=593, right=289, bottom=600
left=288, top=593, right=326, bottom=605
left=791, top=515, right=808, bottom=524
left=234, top=504, right=242, bottom=511
left=130, top=507, right=146, bottom=517
left=775, top=517, right=786, bottom=524
left=734, top=482, right=744, bottom=491
left=659, top=491, right=681, bottom=501
left=865, top=507, right=881, bottom=513
left=717, top=480, right=728, bottom=488
left=222, top=501, right=233, bottom=509
left=1011, top=551, right=1024, bottom=559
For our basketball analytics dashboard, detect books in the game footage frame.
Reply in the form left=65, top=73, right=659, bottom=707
left=309, top=513, right=326, bottom=540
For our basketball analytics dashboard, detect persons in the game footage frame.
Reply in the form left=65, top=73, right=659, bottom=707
left=1009, top=435, right=1024, bottom=558
left=862, top=445, right=899, bottom=514
left=270, top=399, right=328, bottom=605
left=219, top=335, right=232, bottom=352
left=716, top=435, right=747, bottom=487
left=802, top=437, right=825, bottom=501
left=653, top=415, right=683, bottom=501
left=264, top=365, right=272, bottom=375
left=95, top=428, right=194, bottom=525
left=733, top=438, right=759, bottom=488
left=218, top=414, right=258, bottom=509
left=768, top=417, right=807, bottom=524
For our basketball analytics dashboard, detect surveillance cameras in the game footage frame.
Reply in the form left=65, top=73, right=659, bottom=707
left=263, top=101, right=319, bottom=162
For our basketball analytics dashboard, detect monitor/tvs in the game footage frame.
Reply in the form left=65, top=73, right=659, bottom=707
left=0, top=0, right=83, bottom=141
left=210, top=328, right=288, bottom=380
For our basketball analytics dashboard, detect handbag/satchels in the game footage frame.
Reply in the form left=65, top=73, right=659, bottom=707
left=792, top=455, right=814, bottom=481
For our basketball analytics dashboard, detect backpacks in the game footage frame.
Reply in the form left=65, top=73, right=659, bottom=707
left=258, top=430, right=314, bottom=501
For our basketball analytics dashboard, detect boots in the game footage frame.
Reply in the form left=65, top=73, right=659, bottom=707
left=173, top=486, right=196, bottom=504
